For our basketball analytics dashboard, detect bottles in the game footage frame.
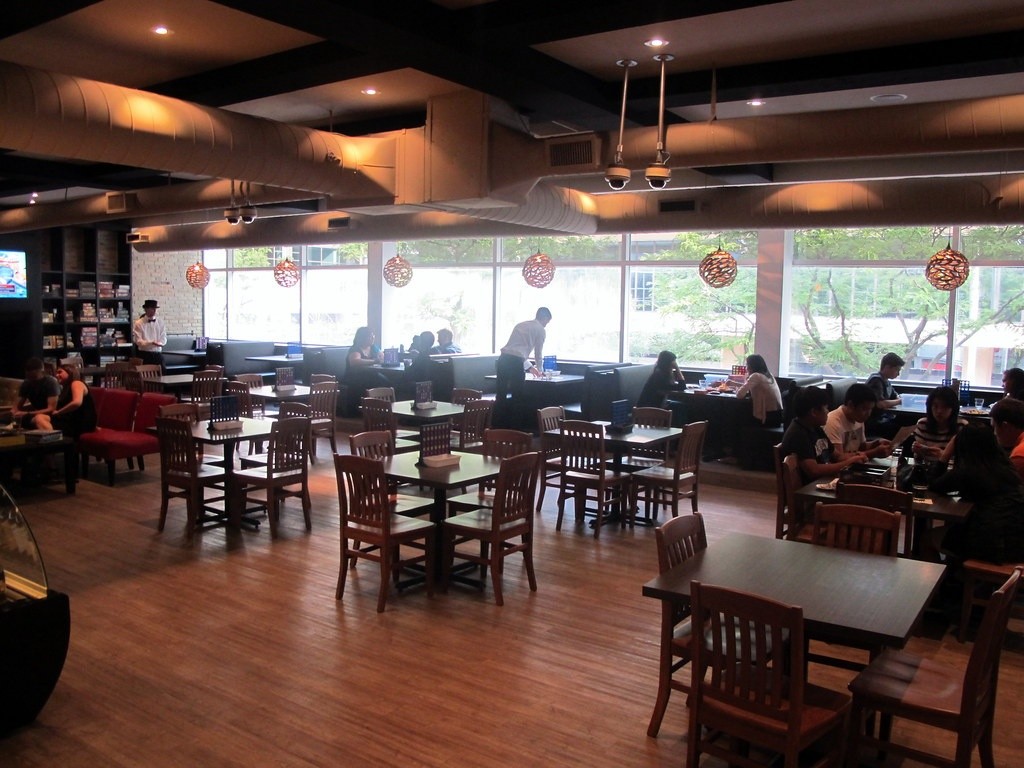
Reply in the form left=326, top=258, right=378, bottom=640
left=533, top=365, right=538, bottom=379
left=896, top=456, right=958, bottom=498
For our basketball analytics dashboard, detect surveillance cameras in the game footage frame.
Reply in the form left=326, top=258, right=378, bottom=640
left=224, top=207, right=242, bottom=225
left=604, top=164, right=631, bottom=190
left=645, top=163, right=671, bottom=189
left=240, top=206, right=257, bottom=224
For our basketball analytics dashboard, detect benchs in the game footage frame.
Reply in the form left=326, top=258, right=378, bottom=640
left=78, top=388, right=178, bottom=487
left=155, top=334, right=853, bottom=428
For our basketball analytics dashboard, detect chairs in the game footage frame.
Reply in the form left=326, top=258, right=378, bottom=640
left=104, top=361, right=1024, bottom=766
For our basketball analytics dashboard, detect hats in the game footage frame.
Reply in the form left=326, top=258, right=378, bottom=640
left=143, top=300, right=159, bottom=308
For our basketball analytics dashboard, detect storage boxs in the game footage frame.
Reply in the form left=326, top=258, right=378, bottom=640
left=23, top=429, right=64, bottom=444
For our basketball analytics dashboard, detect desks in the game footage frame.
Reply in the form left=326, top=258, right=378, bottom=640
left=485, top=372, right=585, bottom=386
left=642, top=533, right=946, bottom=686
left=881, top=394, right=930, bottom=421
left=0, top=428, right=75, bottom=494
left=364, top=363, right=407, bottom=384
left=244, top=354, right=304, bottom=369
left=543, top=420, right=684, bottom=527
left=356, top=399, right=489, bottom=422
left=162, top=349, right=206, bottom=365
left=677, top=383, right=748, bottom=407
left=227, top=386, right=340, bottom=403
left=186, top=417, right=278, bottom=530
left=143, top=374, right=233, bottom=403
left=376, top=449, right=508, bottom=592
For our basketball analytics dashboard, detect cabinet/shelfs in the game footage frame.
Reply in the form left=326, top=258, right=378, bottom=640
left=42, top=270, right=135, bottom=385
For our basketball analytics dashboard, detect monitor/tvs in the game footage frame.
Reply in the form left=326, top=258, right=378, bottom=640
left=0, top=250, right=28, bottom=298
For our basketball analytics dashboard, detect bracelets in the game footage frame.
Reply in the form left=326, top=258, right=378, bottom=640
left=871, top=439, right=879, bottom=448
left=855, top=451, right=860, bottom=456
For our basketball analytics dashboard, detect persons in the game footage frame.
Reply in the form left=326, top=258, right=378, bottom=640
left=10, top=359, right=96, bottom=441
left=637, top=350, right=686, bottom=457
left=716, top=354, right=784, bottom=464
left=492, top=307, right=552, bottom=431
left=911, top=386, right=1024, bottom=564
left=824, top=384, right=893, bottom=461
left=781, top=386, right=868, bottom=485
left=866, top=352, right=916, bottom=458
left=134, top=299, right=167, bottom=376
left=343, top=327, right=392, bottom=388
left=1001, top=368, right=1024, bottom=399
left=404, top=328, right=462, bottom=372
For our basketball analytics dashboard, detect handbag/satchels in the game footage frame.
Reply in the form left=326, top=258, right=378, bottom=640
left=895, top=455, right=935, bottom=492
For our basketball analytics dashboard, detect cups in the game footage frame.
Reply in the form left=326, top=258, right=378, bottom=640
left=542, top=369, right=561, bottom=380
left=974, top=398, right=984, bottom=410
left=699, top=380, right=706, bottom=389
left=900, top=393, right=918, bottom=407
